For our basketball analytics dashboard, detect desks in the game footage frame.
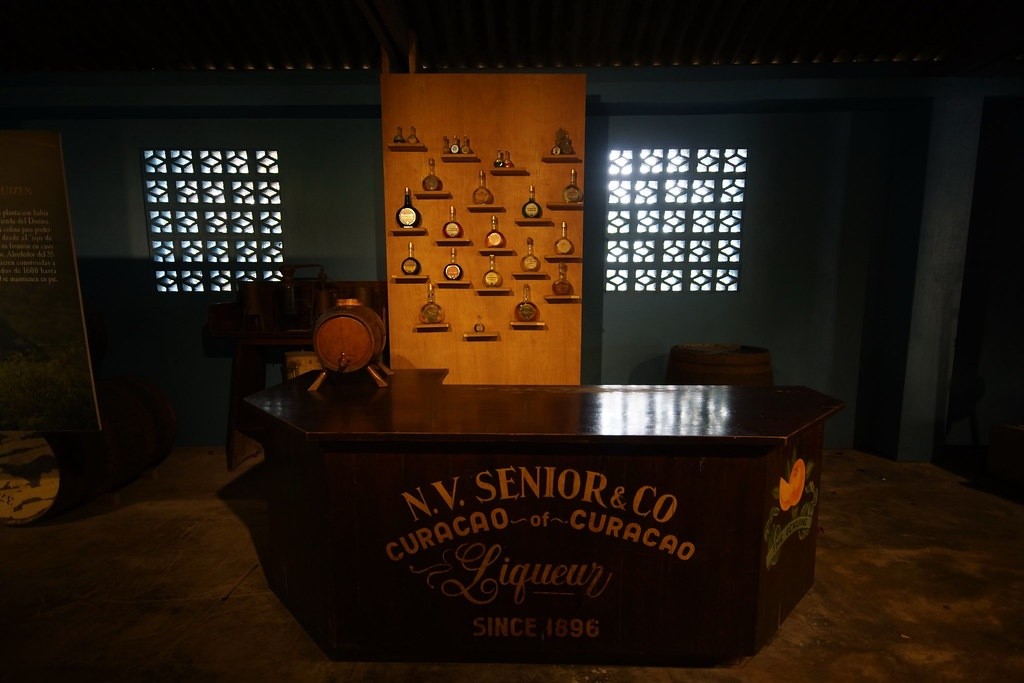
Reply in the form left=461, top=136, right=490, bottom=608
left=230, top=371, right=844, bottom=652
left=204, top=280, right=388, bottom=459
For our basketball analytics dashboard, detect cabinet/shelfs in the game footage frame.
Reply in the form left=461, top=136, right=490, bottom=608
left=388, top=143, right=583, bottom=337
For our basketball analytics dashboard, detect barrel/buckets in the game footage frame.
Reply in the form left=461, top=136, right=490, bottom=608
left=285, top=350, right=320, bottom=380
left=314, top=299, right=387, bottom=375
left=0, top=296, right=178, bottom=527
left=663, top=343, right=774, bottom=385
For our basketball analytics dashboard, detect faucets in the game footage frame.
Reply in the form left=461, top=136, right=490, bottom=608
left=337, top=351, right=350, bottom=371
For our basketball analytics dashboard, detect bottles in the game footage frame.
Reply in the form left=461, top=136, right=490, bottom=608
left=402, top=242, right=420, bottom=275
left=563, top=169, right=583, bottom=201
left=422, top=158, right=442, bottom=191
left=473, top=171, right=493, bottom=205
left=484, top=255, right=502, bottom=287
left=554, top=221, right=574, bottom=255
left=485, top=216, right=505, bottom=248
left=520, top=238, right=541, bottom=271
left=494, top=149, right=515, bottom=168
left=443, top=134, right=471, bottom=154
left=396, top=187, right=421, bottom=228
left=522, top=185, right=542, bottom=218
left=419, top=284, right=443, bottom=323
left=392, top=127, right=419, bottom=144
left=474, top=316, right=485, bottom=331
left=442, top=205, right=463, bottom=238
left=515, top=284, right=539, bottom=321
left=552, top=263, right=573, bottom=295
left=551, top=130, right=572, bottom=154
left=444, top=248, right=462, bottom=280
